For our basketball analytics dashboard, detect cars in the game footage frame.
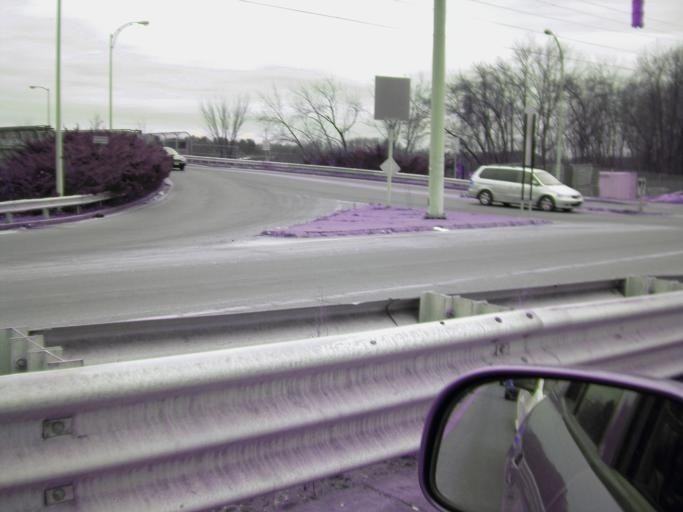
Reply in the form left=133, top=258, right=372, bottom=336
left=488, top=375, right=683, bottom=512
left=160, top=144, right=188, bottom=170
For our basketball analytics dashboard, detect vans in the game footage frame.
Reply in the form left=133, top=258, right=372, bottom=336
left=470, top=162, right=582, bottom=216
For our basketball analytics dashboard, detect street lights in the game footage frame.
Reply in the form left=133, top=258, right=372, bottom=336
left=543, top=28, right=568, bottom=182
left=104, top=17, right=149, bottom=132
left=30, top=84, right=52, bottom=128
